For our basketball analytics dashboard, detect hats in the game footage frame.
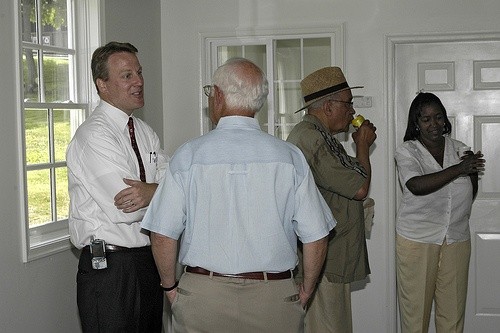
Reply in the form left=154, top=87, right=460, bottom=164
left=294, top=66, right=364, bottom=113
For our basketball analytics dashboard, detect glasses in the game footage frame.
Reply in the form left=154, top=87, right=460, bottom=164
left=328, top=98, right=355, bottom=110
left=203, top=85, right=221, bottom=97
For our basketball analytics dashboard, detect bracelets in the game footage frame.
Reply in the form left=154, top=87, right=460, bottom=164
left=158, top=281, right=179, bottom=292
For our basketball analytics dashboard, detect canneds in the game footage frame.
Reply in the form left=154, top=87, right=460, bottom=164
left=351, top=114, right=365, bottom=131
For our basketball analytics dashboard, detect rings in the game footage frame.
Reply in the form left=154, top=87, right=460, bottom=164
left=130, top=200, right=134, bottom=205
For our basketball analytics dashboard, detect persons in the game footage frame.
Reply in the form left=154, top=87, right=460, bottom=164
left=394, top=93, right=486, bottom=333
left=140, top=57, right=337, bottom=333
left=285, top=67, right=377, bottom=333
left=66, top=41, right=171, bottom=333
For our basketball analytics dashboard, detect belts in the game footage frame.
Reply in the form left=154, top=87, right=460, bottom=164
left=105, top=243, right=149, bottom=253
left=186, top=266, right=295, bottom=281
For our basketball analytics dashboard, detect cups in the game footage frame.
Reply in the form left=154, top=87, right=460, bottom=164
left=458, top=146, right=471, bottom=160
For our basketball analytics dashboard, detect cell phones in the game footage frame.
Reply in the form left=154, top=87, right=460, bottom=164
left=90, top=236, right=107, bottom=269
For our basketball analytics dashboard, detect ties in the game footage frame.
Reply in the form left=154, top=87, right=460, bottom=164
left=128, top=118, right=147, bottom=183
